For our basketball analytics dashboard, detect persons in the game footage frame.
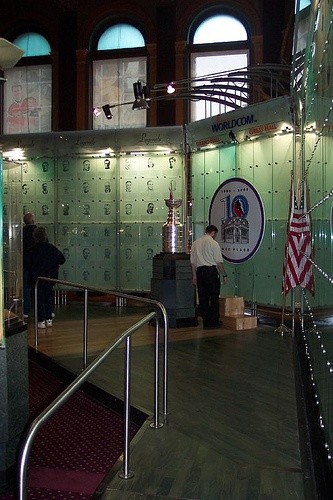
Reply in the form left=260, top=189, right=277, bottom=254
left=190, top=225, right=228, bottom=330
left=16, top=213, right=66, bottom=329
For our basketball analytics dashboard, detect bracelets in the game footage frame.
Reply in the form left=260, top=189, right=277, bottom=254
left=223, top=274, right=227, bottom=278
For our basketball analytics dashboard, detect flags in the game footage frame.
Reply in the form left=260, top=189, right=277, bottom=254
left=302, top=175, right=315, bottom=299
left=281, top=170, right=302, bottom=297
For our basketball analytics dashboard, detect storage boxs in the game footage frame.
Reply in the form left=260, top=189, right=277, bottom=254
left=219, top=296, right=257, bottom=331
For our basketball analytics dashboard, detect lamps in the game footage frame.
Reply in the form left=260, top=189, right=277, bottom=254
left=102, top=63, right=292, bottom=120
left=229, top=130, right=238, bottom=142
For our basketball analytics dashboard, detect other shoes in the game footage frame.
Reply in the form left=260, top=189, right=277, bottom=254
left=38, top=320, right=46, bottom=329
left=46, top=319, right=52, bottom=327
left=214, top=321, right=224, bottom=328
